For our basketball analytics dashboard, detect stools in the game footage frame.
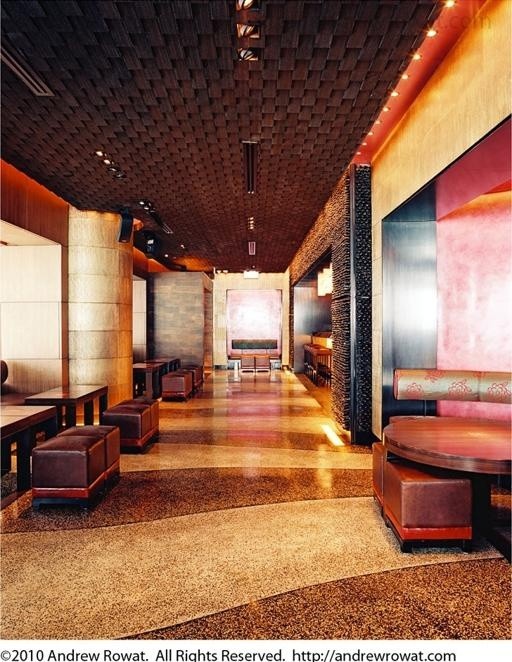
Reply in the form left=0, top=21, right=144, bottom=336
left=161, top=365, right=204, bottom=402
left=302, top=343, right=332, bottom=388
left=371, top=441, right=474, bottom=554
left=241, top=353, right=270, bottom=373
left=32, top=397, right=161, bottom=511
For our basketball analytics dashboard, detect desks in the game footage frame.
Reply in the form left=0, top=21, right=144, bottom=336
left=132, top=358, right=181, bottom=398
left=1, top=383, right=109, bottom=493
left=382, top=417, right=511, bottom=476
